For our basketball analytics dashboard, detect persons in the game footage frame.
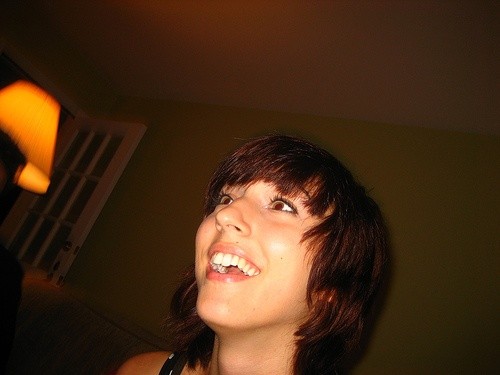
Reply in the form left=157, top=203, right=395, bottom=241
left=118, top=134, right=386, bottom=375
